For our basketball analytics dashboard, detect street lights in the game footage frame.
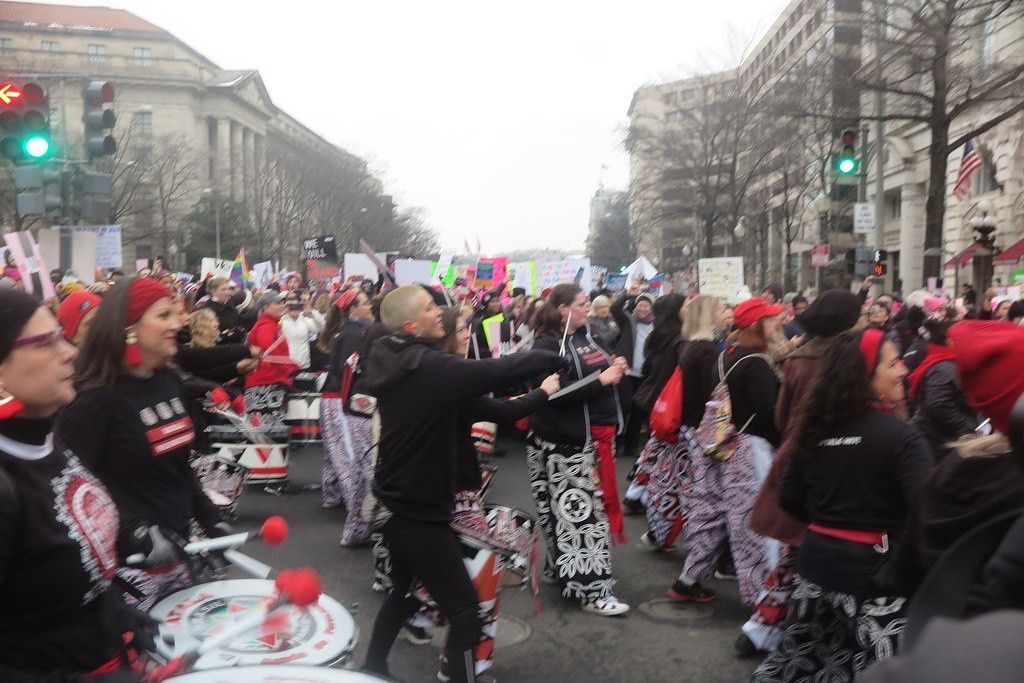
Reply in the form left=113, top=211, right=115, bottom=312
left=203, top=187, right=224, bottom=262
left=732, top=209, right=770, bottom=296
left=966, top=198, right=1003, bottom=321
left=923, top=248, right=960, bottom=300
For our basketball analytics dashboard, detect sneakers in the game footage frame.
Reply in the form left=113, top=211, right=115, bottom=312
left=618, top=497, right=645, bottom=512
left=580, top=593, right=630, bottom=616
left=400, top=622, right=433, bottom=644
left=713, top=565, right=736, bottom=579
left=667, top=579, right=717, bottom=601
left=640, top=531, right=676, bottom=552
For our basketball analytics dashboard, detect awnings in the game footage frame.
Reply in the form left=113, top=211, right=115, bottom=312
left=945, top=242, right=986, bottom=267
left=991, top=240, right=1024, bottom=265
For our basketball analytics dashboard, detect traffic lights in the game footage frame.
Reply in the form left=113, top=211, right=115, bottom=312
left=847, top=248, right=872, bottom=277
left=0, top=82, right=23, bottom=162
left=873, top=249, right=889, bottom=277
left=72, top=169, right=115, bottom=225
left=12, top=167, right=64, bottom=223
left=80, top=75, right=117, bottom=159
left=838, top=128, right=858, bottom=175
left=20, top=83, right=56, bottom=164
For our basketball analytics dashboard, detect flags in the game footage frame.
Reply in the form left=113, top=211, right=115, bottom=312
left=953, top=138, right=981, bottom=200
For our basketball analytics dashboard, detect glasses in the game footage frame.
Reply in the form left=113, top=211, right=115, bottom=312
left=8, top=326, right=64, bottom=356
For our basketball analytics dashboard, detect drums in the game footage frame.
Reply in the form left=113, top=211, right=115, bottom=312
left=482, top=502, right=539, bottom=590
left=467, top=419, right=498, bottom=458
left=449, top=461, right=498, bottom=507
left=204, top=423, right=291, bottom=485
left=411, top=519, right=517, bottom=625
left=283, top=393, right=329, bottom=445
left=185, top=448, right=250, bottom=510
left=138, top=575, right=361, bottom=672
left=160, top=663, right=402, bottom=683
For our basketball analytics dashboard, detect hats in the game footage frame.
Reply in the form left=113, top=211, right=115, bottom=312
left=734, top=298, right=786, bottom=329
left=794, top=289, right=862, bottom=338
left=286, top=271, right=303, bottom=286
left=946, top=318, right=1024, bottom=435
left=924, top=297, right=946, bottom=313
left=635, top=291, right=655, bottom=305
left=54, top=282, right=86, bottom=302
left=991, top=295, right=1010, bottom=312
left=481, top=291, right=495, bottom=306
left=761, top=281, right=782, bottom=299
left=255, top=289, right=288, bottom=308
left=56, top=289, right=103, bottom=340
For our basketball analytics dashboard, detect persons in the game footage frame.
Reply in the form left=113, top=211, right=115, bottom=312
left=363, top=287, right=561, bottom=683
left=0, top=252, right=784, bottom=610
left=737, top=275, right=1023, bottom=682
left=60, top=279, right=227, bottom=607
left=1, top=289, right=201, bottom=683
left=526, top=283, right=630, bottom=616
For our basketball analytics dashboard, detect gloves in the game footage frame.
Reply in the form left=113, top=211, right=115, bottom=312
left=89, top=587, right=176, bottom=652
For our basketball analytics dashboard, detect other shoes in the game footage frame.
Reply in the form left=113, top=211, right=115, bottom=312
left=321, top=502, right=333, bottom=508
left=339, top=538, right=351, bottom=545
left=437, top=659, right=451, bottom=681
left=733, top=631, right=760, bottom=657
left=265, top=482, right=287, bottom=494
left=371, top=582, right=385, bottom=591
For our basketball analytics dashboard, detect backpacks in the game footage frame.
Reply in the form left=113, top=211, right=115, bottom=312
left=650, top=366, right=682, bottom=444
left=694, top=349, right=775, bottom=460
